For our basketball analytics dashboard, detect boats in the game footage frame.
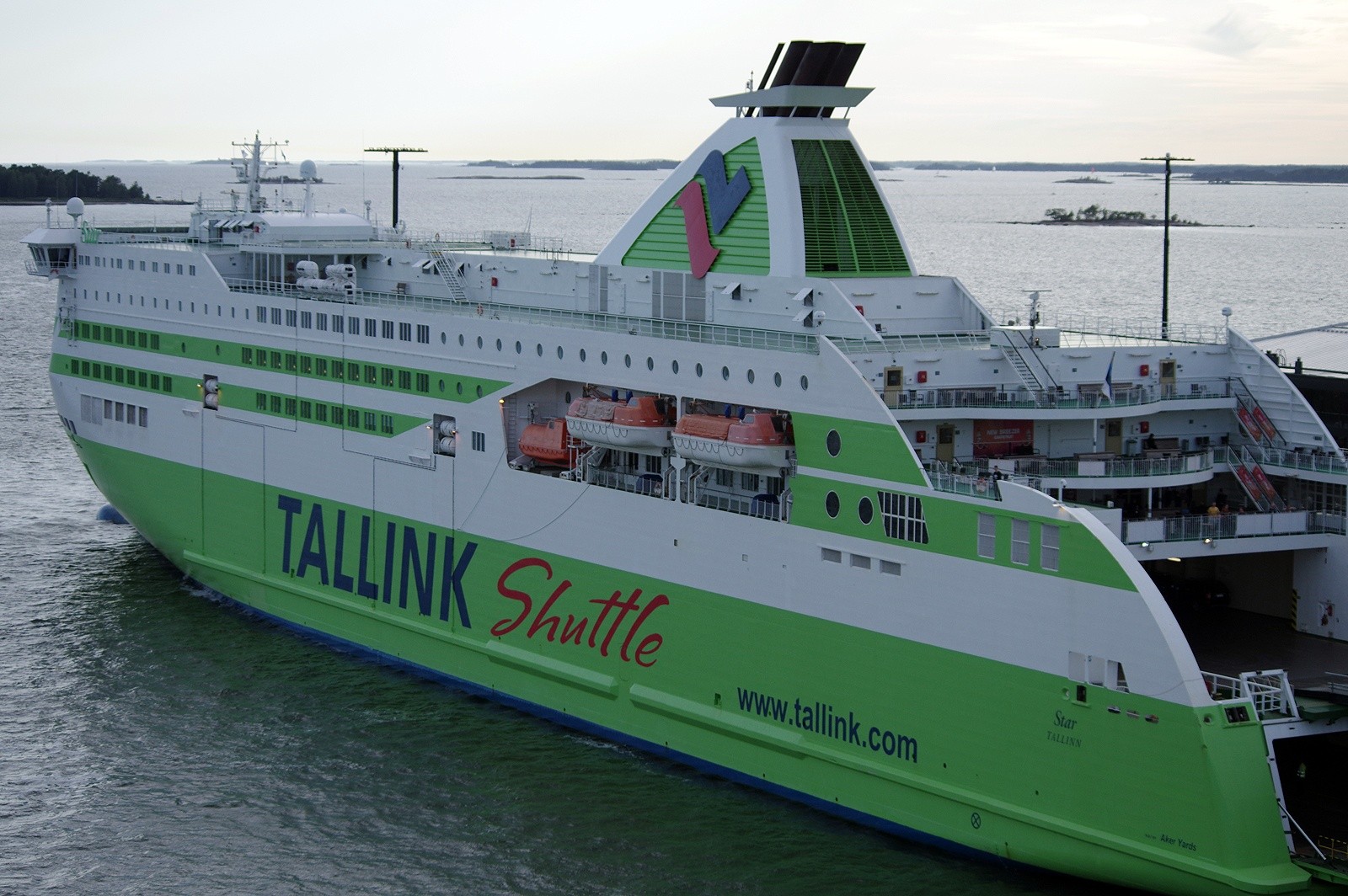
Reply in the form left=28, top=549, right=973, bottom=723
left=19, top=37, right=1348, bottom=896
left=519, top=417, right=592, bottom=468
left=565, top=396, right=675, bottom=457
left=671, top=411, right=796, bottom=479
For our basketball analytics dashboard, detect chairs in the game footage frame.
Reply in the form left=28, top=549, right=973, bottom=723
left=1057, top=385, right=1071, bottom=404
left=391, top=283, right=406, bottom=295
left=1286, top=447, right=1336, bottom=466
left=899, top=392, right=1007, bottom=408
left=1166, top=383, right=1211, bottom=398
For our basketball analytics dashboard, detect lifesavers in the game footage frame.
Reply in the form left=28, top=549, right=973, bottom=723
left=977, top=479, right=985, bottom=492
left=435, top=233, right=439, bottom=242
left=655, top=482, right=662, bottom=496
left=50, top=269, right=59, bottom=274
left=477, top=305, right=483, bottom=316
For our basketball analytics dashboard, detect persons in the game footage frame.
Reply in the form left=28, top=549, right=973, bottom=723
left=1131, top=489, right=1293, bottom=536
left=478, top=303, right=483, bottom=316
left=1147, top=432, right=1157, bottom=450
left=993, top=466, right=1001, bottom=501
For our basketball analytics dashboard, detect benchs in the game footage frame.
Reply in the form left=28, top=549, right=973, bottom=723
left=1079, top=383, right=1132, bottom=397
left=1143, top=438, right=1179, bottom=449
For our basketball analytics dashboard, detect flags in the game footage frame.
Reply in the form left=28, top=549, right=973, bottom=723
left=492, top=278, right=497, bottom=287
left=1101, top=358, right=1112, bottom=405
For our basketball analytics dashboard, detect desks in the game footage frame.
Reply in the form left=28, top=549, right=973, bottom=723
left=1142, top=450, right=1182, bottom=458
left=1004, top=455, right=1046, bottom=460
left=1074, top=451, right=1115, bottom=460
